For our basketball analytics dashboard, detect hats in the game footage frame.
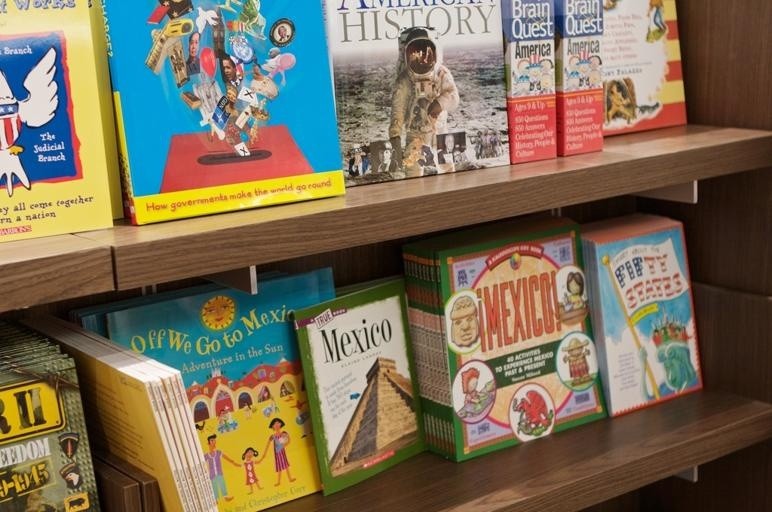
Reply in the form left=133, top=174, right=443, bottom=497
left=450, top=296, right=475, bottom=320
left=561, top=338, right=589, bottom=352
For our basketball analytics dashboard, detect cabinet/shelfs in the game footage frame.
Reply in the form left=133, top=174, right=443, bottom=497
left=0, top=123, right=772, bottom=512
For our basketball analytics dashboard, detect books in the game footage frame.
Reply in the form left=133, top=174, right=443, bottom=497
left=1, top=210, right=706, bottom=512
left=1, top=0, right=688, bottom=243
